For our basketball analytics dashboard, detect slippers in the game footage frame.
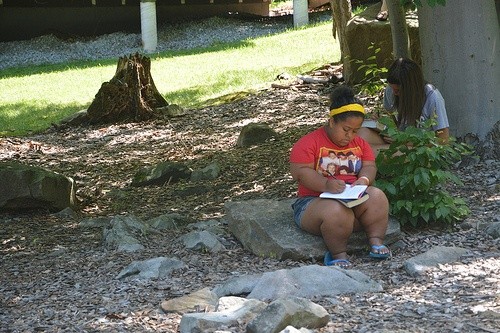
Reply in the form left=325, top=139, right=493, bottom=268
left=370, top=245, right=390, bottom=258
left=324, top=251, right=352, bottom=270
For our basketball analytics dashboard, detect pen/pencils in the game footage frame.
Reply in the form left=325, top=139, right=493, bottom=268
left=326, top=171, right=340, bottom=180
left=378, top=110, right=379, bottom=118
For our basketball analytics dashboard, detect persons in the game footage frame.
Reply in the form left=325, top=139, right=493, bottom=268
left=356, top=58, right=450, bottom=165
left=289, top=87, right=391, bottom=270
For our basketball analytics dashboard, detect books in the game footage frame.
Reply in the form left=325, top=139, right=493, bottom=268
left=319, top=183, right=369, bottom=208
left=362, top=120, right=387, bottom=132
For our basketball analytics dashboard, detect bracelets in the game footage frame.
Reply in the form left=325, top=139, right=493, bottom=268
left=360, top=176, right=369, bottom=184
